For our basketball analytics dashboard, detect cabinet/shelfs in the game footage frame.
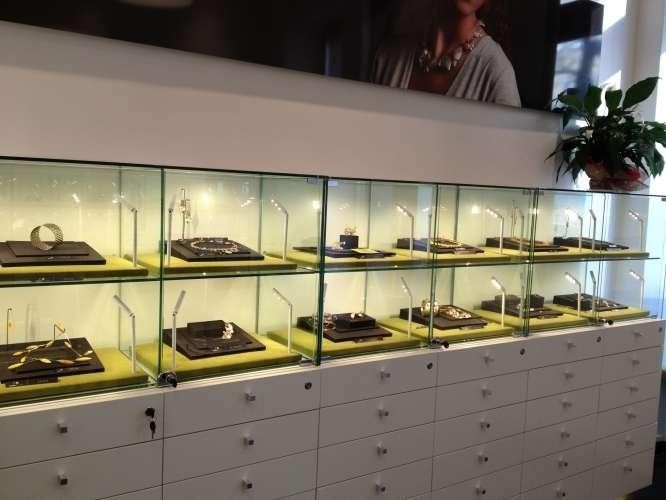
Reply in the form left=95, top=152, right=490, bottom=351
left=1, top=314, right=663, bottom=500
left=0, top=156, right=666, bottom=408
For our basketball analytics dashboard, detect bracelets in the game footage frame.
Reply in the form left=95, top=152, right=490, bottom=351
left=30, top=223, right=63, bottom=251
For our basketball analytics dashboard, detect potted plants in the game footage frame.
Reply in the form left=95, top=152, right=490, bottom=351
left=547, top=75, right=666, bottom=189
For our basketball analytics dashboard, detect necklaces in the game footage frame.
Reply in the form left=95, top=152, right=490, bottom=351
left=415, top=19, right=486, bottom=73
left=190, top=240, right=239, bottom=254
left=430, top=239, right=463, bottom=248
left=8, top=321, right=93, bottom=371
left=512, top=237, right=548, bottom=246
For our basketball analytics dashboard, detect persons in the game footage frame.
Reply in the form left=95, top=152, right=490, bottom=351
left=366, top=0, right=522, bottom=108
